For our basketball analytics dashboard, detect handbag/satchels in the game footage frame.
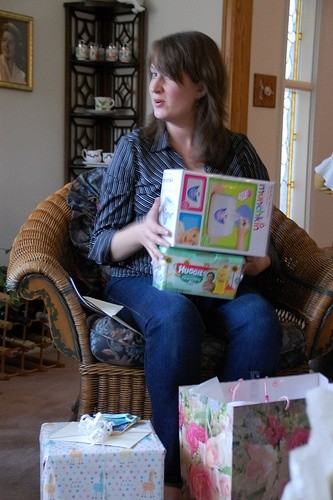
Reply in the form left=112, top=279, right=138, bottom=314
left=178, top=372, right=329, bottom=500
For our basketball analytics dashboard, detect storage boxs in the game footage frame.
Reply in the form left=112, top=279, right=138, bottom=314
left=151, top=246, right=248, bottom=301
left=158, top=169, right=275, bottom=258
left=39, top=419, right=167, bottom=500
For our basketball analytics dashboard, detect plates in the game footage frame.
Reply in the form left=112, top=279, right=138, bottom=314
left=81, top=161, right=110, bottom=167
left=86, top=109, right=116, bottom=115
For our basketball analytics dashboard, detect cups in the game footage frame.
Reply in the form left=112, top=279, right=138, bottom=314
left=75, top=39, right=132, bottom=62
left=103, top=153, right=115, bottom=163
left=93, top=96, right=114, bottom=111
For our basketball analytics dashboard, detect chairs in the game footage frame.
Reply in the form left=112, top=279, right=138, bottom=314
left=3, top=179, right=333, bottom=423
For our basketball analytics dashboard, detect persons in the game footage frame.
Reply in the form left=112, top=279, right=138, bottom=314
left=88, top=31, right=271, bottom=485
left=202, top=272, right=215, bottom=293
left=0, top=21, right=26, bottom=84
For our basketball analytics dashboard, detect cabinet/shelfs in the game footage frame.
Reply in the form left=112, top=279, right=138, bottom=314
left=63, top=0, right=147, bottom=184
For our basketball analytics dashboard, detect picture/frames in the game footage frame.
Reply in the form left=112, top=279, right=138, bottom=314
left=0, top=11, right=34, bottom=93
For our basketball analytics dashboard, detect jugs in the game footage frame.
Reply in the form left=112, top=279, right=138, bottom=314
left=81, top=149, right=103, bottom=162
